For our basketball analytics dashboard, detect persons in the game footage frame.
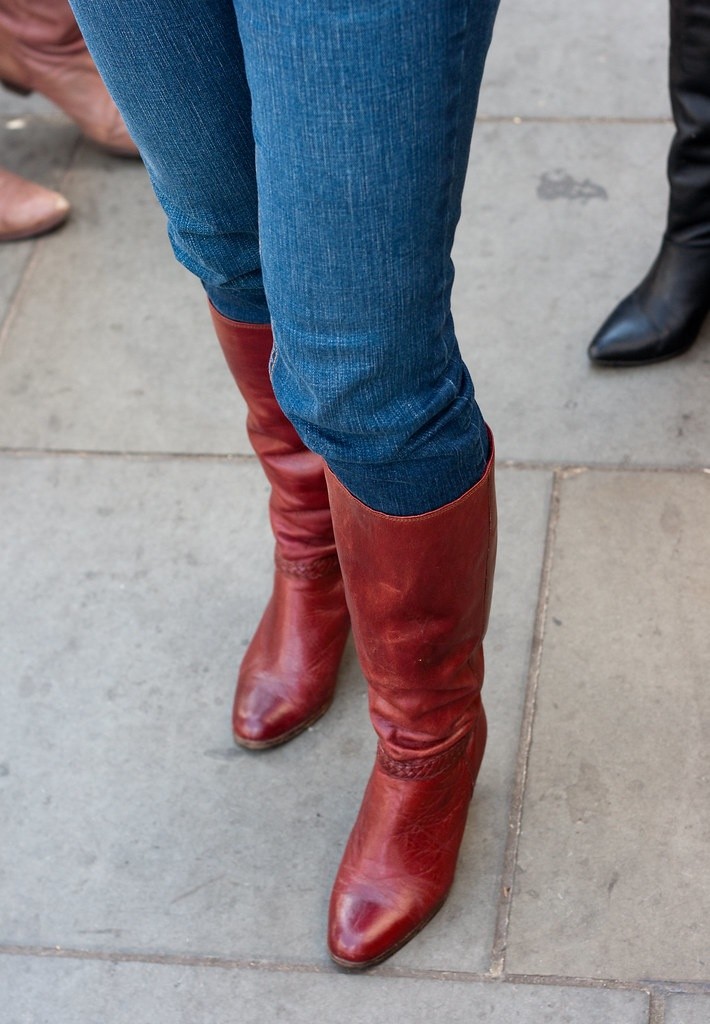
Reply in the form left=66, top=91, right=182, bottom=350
left=0, top=0, right=143, bottom=241
left=75, top=0, right=501, bottom=971
left=588, top=0, right=710, bottom=368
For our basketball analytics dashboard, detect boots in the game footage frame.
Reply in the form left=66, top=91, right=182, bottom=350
left=207, top=296, right=351, bottom=750
left=0, top=0, right=141, bottom=156
left=324, top=420, right=497, bottom=968
left=0, top=165, right=69, bottom=241
left=589, top=0, right=710, bottom=366
